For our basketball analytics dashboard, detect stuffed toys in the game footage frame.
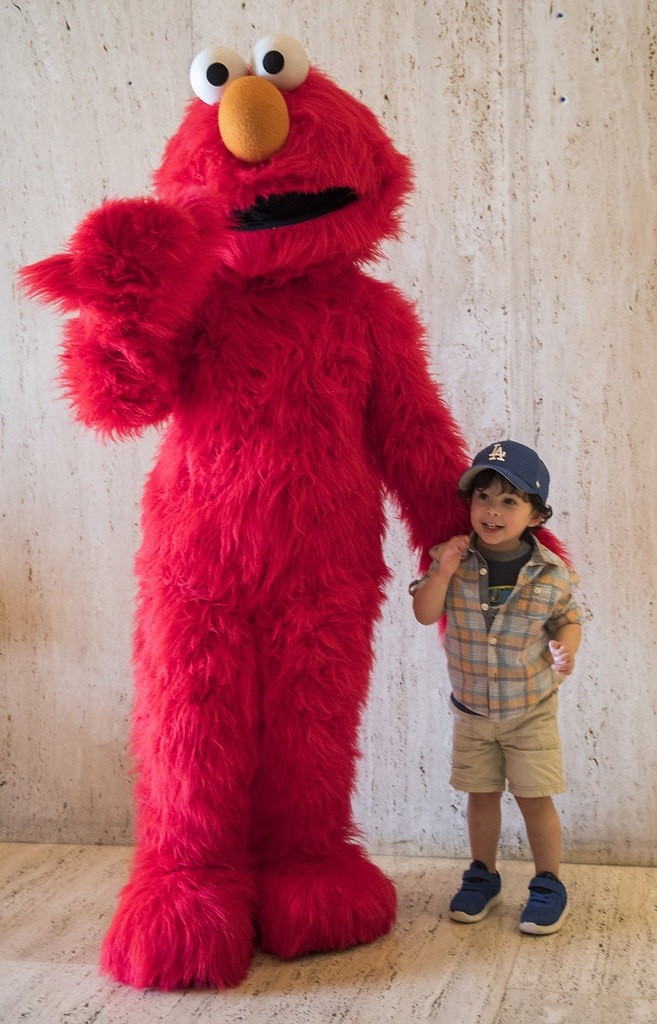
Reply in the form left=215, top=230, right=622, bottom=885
left=16, top=34, right=578, bottom=990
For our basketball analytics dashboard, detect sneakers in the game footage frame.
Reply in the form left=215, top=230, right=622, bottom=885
left=447, top=860, right=503, bottom=923
left=519, top=871, right=570, bottom=934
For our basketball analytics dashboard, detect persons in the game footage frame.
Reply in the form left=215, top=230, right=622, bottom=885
left=403, top=439, right=579, bottom=938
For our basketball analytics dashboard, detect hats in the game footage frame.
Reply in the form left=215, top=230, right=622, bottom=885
left=459, top=440, right=551, bottom=504
